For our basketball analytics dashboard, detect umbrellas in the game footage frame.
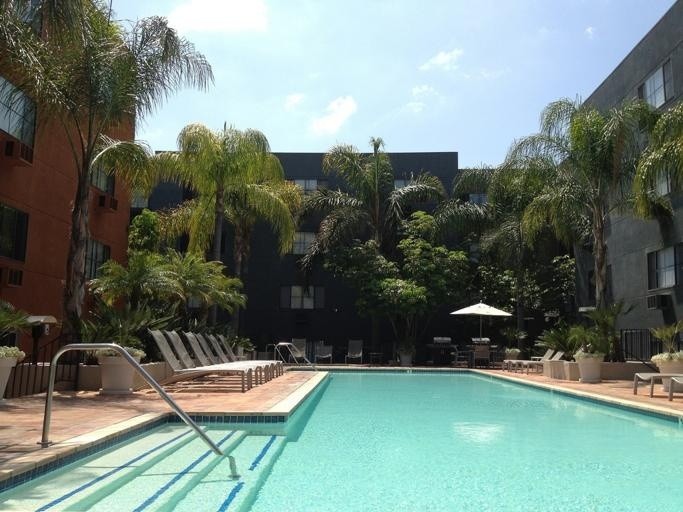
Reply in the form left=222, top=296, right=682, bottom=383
left=447, top=299, right=513, bottom=346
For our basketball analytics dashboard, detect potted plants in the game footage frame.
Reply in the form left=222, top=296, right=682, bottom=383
left=565, top=324, right=605, bottom=386
left=94, top=340, right=148, bottom=390
left=642, top=318, right=681, bottom=390
left=0, top=343, right=25, bottom=405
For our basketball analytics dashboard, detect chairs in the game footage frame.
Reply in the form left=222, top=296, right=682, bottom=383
left=144, top=327, right=366, bottom=391
left=502, top=347, right=567, bottom=377
left=632, top=367, right=682, bottom=401
